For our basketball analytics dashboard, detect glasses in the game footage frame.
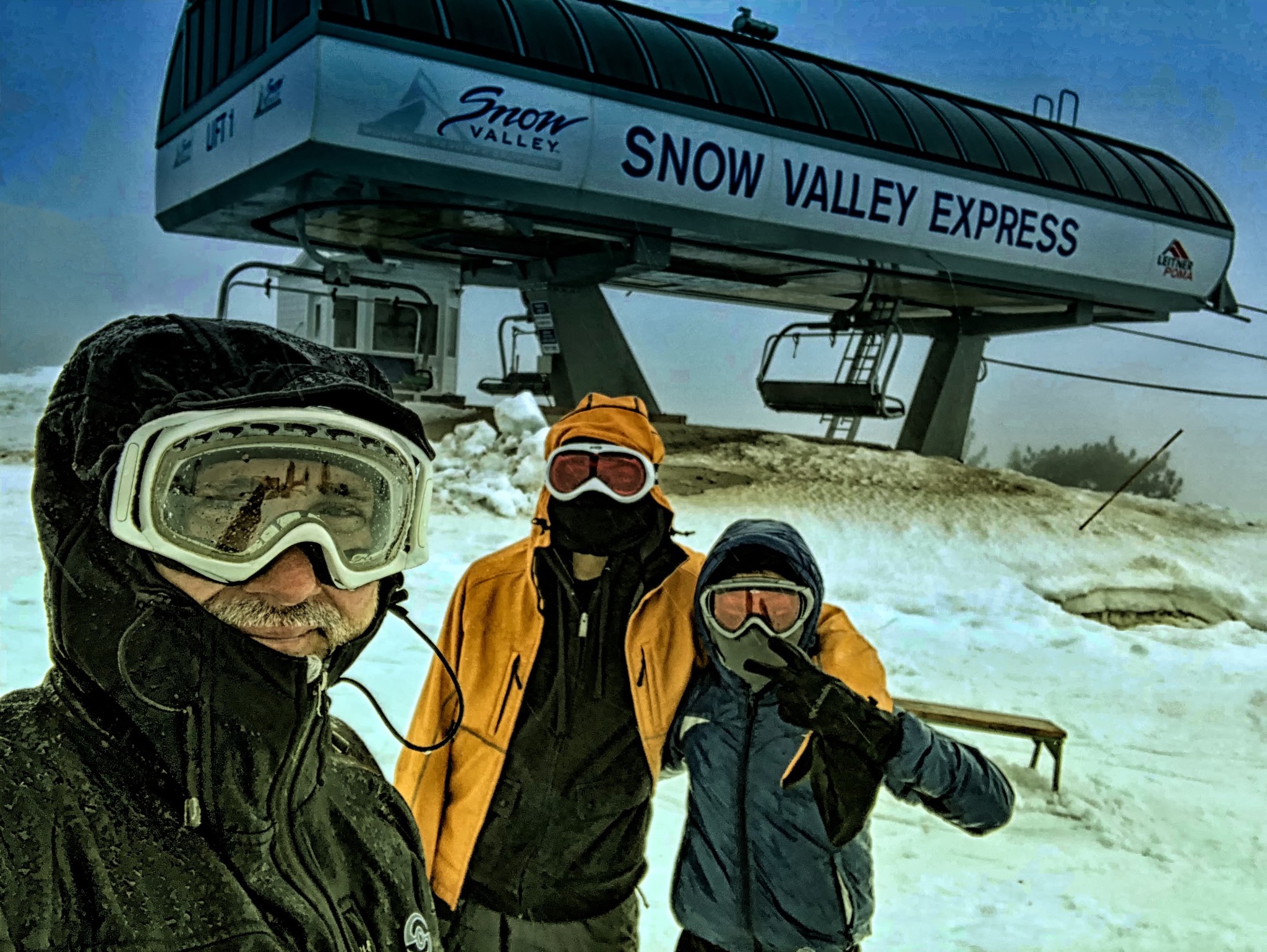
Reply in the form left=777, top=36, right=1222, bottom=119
left=109, top=409, right=436, bottom=589
left=545, top=442, right=655, bottom=502
left=699, top=577, right=817, bottom=642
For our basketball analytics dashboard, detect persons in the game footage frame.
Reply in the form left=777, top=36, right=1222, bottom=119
left=389, top=389, right=894, bottom=952
left=660, top=518, right=1016, bottom=952
left=0, top=310, right=457, bottom=952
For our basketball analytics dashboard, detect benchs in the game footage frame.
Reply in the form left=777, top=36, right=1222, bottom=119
left=891, top=697, right=1068, bottom=793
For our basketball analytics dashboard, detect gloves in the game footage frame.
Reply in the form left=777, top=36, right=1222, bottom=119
left=741, top=635, right=904, bottom=767
